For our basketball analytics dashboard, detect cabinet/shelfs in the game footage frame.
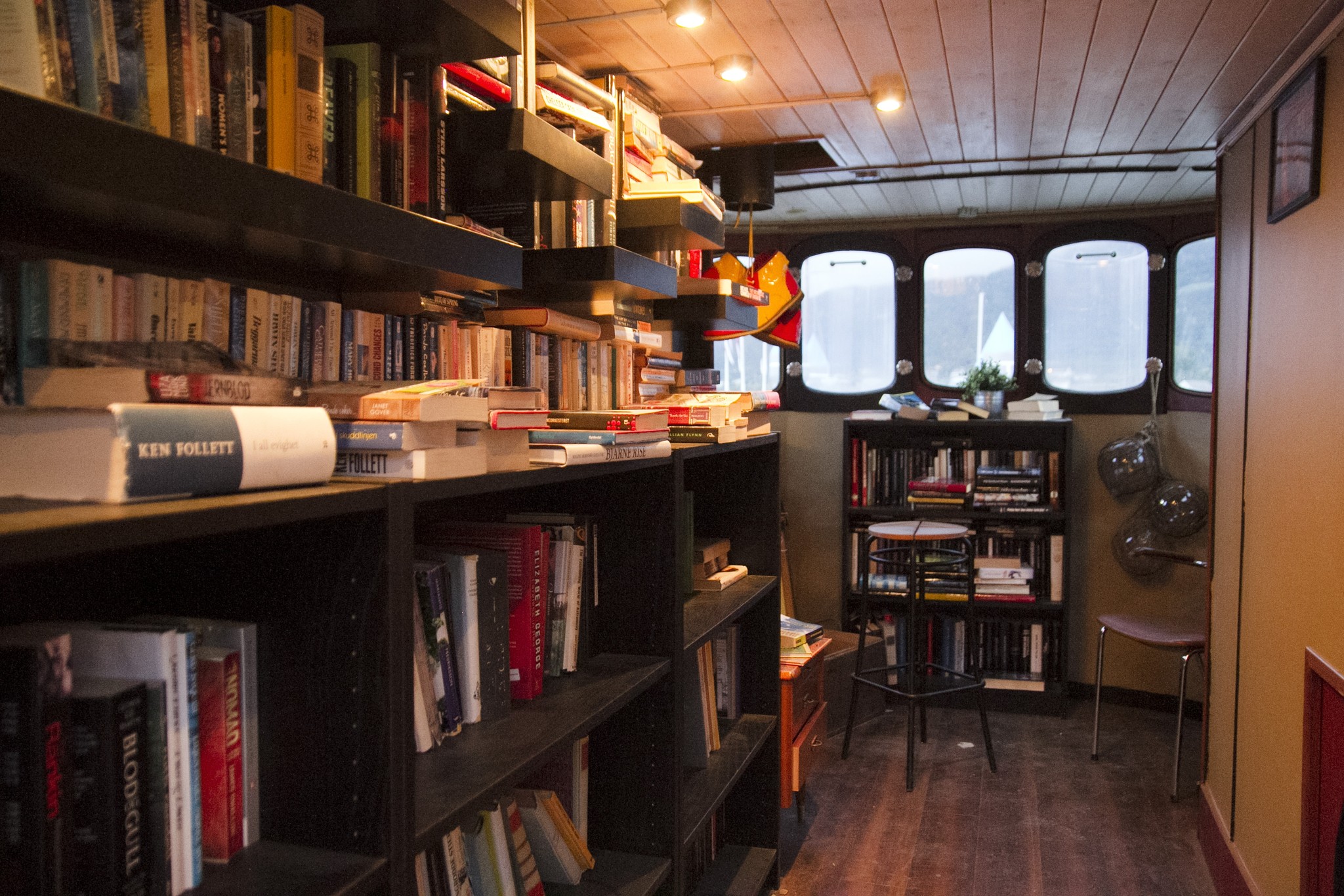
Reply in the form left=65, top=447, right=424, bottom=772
left=0, top=0, right=792, bottom=896
left=838, top=417, right=1073, bottom=717
left=777, top=637, right=829, bottom=809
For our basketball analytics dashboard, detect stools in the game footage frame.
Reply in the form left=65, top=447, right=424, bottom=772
left=841, top=521, right=996, bottom=793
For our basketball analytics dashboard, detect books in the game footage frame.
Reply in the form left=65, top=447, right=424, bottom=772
left=852, top=391, right=1063, bottom=692
left=0, top=0, right=725, bottom=275
left=0, top=258, right=782, bottom=505
left=0, top=614, right=262, bottom=896
left=781, top=614, right=832, bottom=666
left=412, top=735, right=596, bottom=896
left=683, top=626, right=736, bottom=758
left=412, top=512, right=599, bottom=754
left=692, top=798, right=726, bottom=881
left=693, top=538, right=748, bottom=591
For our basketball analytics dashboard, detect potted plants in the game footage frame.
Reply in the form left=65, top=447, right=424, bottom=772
left=962, top=361, right=1018, bottom=418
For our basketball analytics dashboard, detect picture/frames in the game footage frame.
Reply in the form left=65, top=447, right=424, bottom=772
left=1266, top=54, right=1325, bottom=225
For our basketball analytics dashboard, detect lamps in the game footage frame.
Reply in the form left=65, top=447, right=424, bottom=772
left=714, top=55, right=754, bottom=81
left=666, top=0, right=712, bottom=27
left=872, top=88, right=906, bottom=111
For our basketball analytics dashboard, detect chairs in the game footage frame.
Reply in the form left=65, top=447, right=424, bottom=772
left=1091, top=546, right=1209, bottom=800
